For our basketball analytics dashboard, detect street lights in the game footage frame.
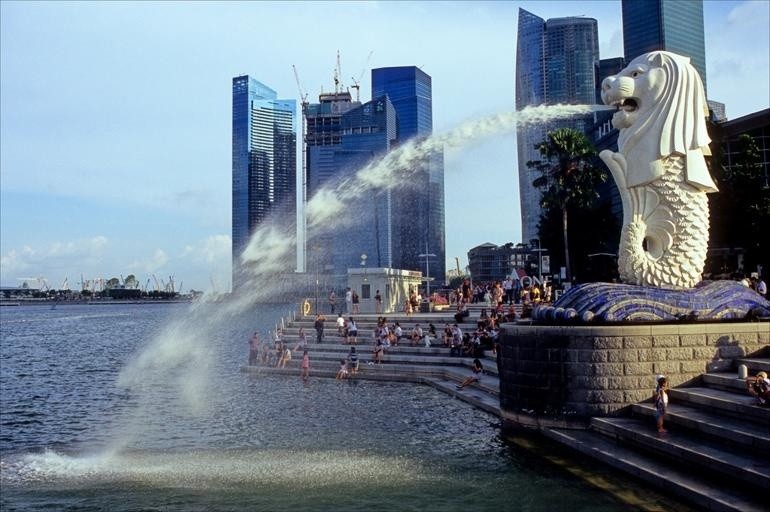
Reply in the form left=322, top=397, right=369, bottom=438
left=313, top=242, right=322, bottom=317
left=418, top=242, right=438, bottom=297
left=531, top=239, right=549, bottom=279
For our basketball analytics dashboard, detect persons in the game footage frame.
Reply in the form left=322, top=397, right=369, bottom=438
left=746, top=371, right=770, bottom=408
left=655, top=379, right=669, bottom=433
left=703, top=263, right=767, bottom=300
